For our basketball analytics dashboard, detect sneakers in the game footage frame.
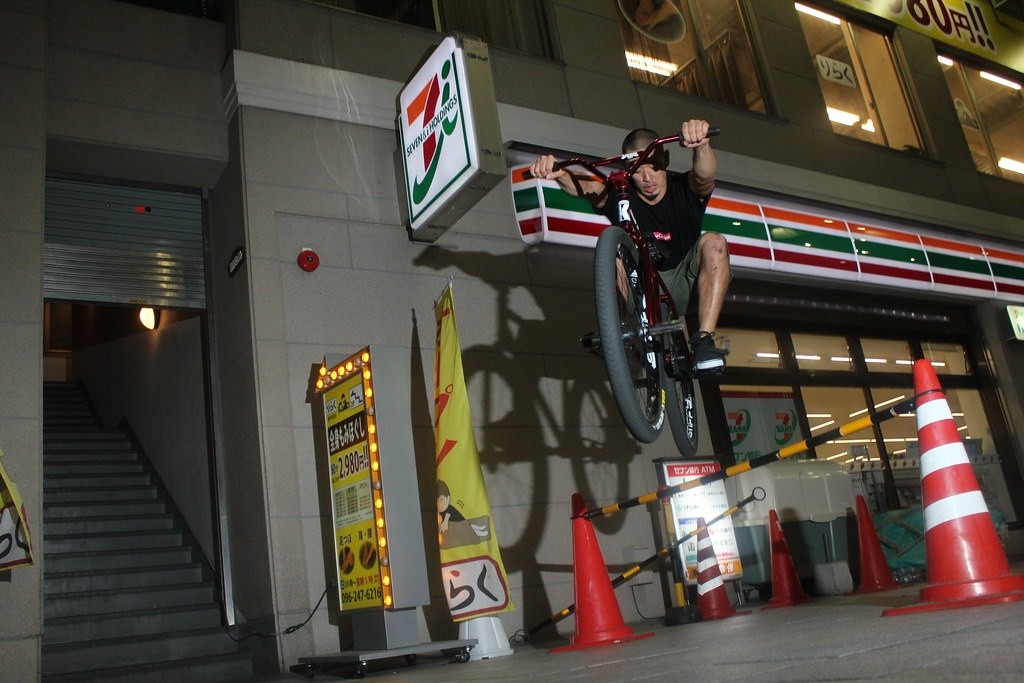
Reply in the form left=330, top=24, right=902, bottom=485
left=689, top=330, right=725, bottom=370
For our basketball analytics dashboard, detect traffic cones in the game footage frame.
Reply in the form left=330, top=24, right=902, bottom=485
left=844, top=493, right=914, bottom=598
left=691, top=518, right=754, bottom=626
left=879, top=359, right=1023, bottom=617
left=548, top=493, right=656, bottom=656
left=760, top=510, right=815, bottom=613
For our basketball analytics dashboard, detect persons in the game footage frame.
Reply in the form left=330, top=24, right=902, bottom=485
left=527, top=116, right=733, bottom=370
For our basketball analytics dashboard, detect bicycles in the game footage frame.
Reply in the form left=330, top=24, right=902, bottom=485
left=522, top=125, right=730, bottom=459
